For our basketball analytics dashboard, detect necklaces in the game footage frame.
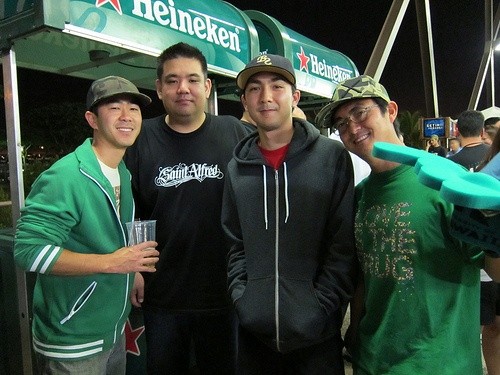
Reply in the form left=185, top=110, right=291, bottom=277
left=465, top=142, right=483, bottom=147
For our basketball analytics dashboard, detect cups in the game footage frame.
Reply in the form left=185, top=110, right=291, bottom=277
left=125, top=219, right=157, bottom=270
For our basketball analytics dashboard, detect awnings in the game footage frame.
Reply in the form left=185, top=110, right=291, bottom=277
left=0, top=0, right=361, bottom=110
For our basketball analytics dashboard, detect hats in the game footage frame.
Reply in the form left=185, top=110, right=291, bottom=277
left=86, top=76, right=152, bottom=109
left=314, top=75, right=391, bottom=128
left=235, top=54, right=296, bottom=90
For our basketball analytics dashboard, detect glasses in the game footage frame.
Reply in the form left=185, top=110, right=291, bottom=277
left=331, top=104, right=380, bottom=136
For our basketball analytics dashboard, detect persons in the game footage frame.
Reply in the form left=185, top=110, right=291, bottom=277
left=220, top=54, right=500, bottom=375
left=123, top=40, right=251, bottom=375
left=12, top=75, right=160, bottom=375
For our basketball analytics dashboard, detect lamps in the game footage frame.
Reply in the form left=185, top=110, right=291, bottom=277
left=89, top=50, right=158, bottom=69
